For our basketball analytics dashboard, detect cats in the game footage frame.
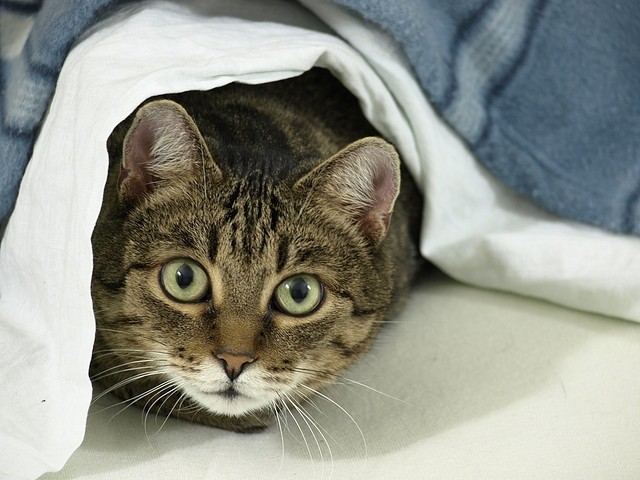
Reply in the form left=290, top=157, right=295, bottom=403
left=88, top=67, right=421, bottom=478
